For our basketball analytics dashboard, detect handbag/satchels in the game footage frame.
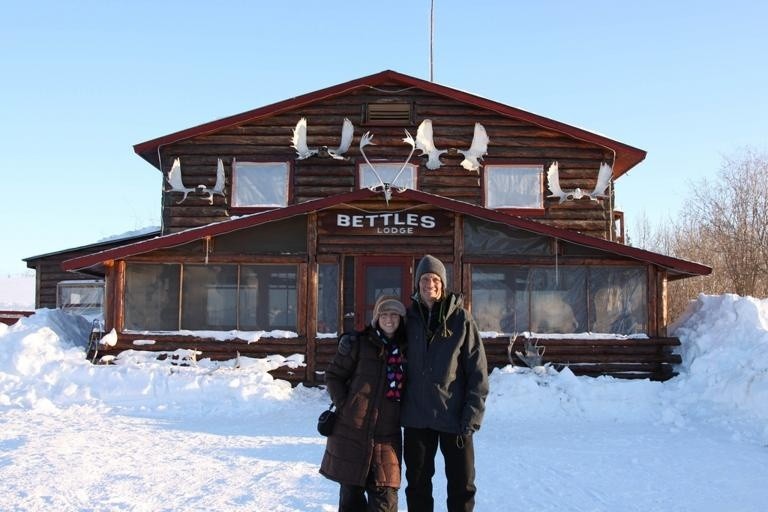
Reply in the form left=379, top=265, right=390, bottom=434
left=317, top=410, right=335, bottom=436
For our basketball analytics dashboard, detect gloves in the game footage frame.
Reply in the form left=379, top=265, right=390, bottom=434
left=338, top=335, right=356, bottom=356
left=456, top=429, right=473, bottom=448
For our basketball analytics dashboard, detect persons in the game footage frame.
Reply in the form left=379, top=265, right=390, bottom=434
left=318, top=292, right=409, bottom=512
left=338, top=254, right=488, bottom=512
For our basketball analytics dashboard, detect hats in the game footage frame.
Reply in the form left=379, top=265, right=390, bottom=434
left=371, top=295, right=406, bottom=328
left=415, top=255, right=447, bottom=289
